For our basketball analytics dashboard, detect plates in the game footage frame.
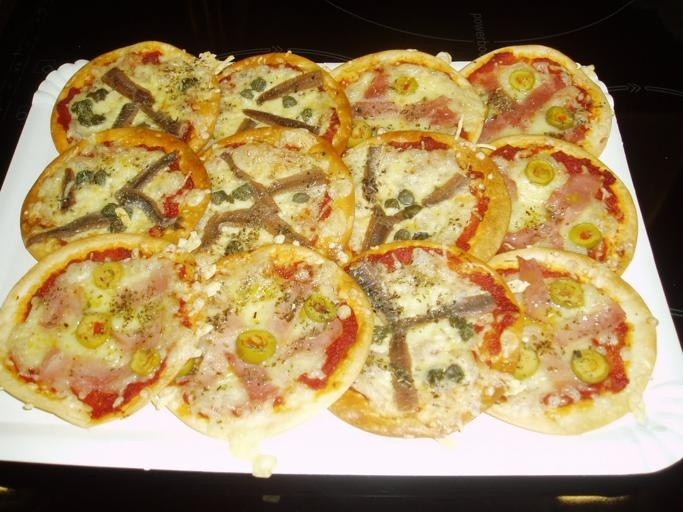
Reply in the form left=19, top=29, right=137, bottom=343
left=1, top=59, right=683, bottom=475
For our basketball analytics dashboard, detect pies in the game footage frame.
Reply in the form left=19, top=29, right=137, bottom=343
left=328, top=49, right=486, bottom=144
left=326, top=240, right=522, bottom=437
left=49, top=40, right=223, bottom=161
left=155, top=240, right=374, bottom=440
left=21, top=127, right=212, bottom=264
left=187, top=127, right=355, bottom=259
left=447, top=44, right=615, bottom=157
left=483, top=133, right=639, bottom=279
left=0, top=229, right=205, bottom=425
left=208, top=51, right=352, bottom=163
left=341, top=130, right=512, bottom=267
left=472, top=249, right=658, bottom=435
